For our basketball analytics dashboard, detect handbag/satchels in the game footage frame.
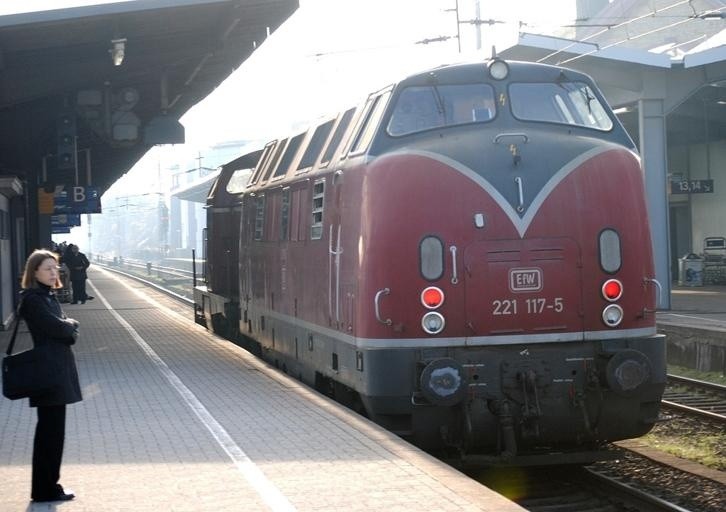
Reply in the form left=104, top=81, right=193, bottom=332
left=1, top=348, right=54, bottom=402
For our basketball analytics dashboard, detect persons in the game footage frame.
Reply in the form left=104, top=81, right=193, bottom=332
left=51, top=241, right=94, bottom=305
left=16, top=248, right=83, bottom=503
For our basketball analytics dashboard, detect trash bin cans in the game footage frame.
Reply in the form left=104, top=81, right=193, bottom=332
left=54, top=263, right=72, bottom=303
left=677, top=253, right=705, bottom=288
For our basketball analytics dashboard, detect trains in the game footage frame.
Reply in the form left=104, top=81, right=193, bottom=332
left=190, top=45, right=669, bottom=470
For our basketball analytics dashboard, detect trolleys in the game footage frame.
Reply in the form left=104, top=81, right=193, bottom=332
left=700, top=236, right=725, bottom=286
left=52, top=268, right=71, bottom=304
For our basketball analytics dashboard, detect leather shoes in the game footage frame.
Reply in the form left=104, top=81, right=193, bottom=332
left=29, top=483, right=76, bottom=503
